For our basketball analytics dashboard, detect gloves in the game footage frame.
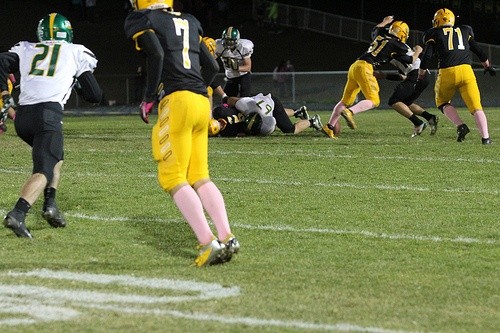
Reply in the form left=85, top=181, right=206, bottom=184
left=0, top=94, right=17, bottom=120
left=139, top=99, right=154, bottom=123
left=230, top=58, right=239, bottom=72
left=484, top=66, right=496, bottom=78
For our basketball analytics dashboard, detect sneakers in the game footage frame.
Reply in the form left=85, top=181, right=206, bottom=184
left=218, top=235, right=240, bottom=262
left=340, top=108, right=356, bottom=129
left=428, top=115, right=439, bottom=136
left=482, top=138, right=493, bottom=145
left=312, top=114, right=323, bottom=131
left=43, top=207, right=65, bottom=228
left=299, top=105, right=309, bottom=120
left=412, top=123, right=427, bottom=137
left=3, top=210, right=32, bottom=239
left=193, top=237, right=224, bottom=267
left=456, top=124, right=469, bottom=142
left=322, top=125, right=335, bottom=139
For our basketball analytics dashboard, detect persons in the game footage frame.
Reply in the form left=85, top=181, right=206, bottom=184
left=371, top=27, right=439, bottom=140
left=207, top=103, right=248, bottom=137
left=211, top=27, right=254, bottom=106
left=157, top=37, right=228, bottom=137
left=123, top=0, right=241, bottom=267
left=321, top=15, right=423, bottom=139
left=414, top=8, right=496, bottom=146
left=276, top=60, right=293, bottom=97
left=0, top=71, right=16, bottom=134
left=227, top=93, right=325, bottom=137
left=0, top=12, right=104, bottom=238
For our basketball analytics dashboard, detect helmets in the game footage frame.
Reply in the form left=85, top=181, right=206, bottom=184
left=245, top=112, right=261, bottom=131
left=203, top=37, right=216, bottom=56
left=432, top=8, right=456, bottom=27
left=207, top=118, right=220, bottom=137
left=130, top=0, right=173, bottom=12
left=222, top=27, right=240, bottom=48
left=36, top=12, right=73, bottom=43
left=388, top=20, right=409, bottom=44
